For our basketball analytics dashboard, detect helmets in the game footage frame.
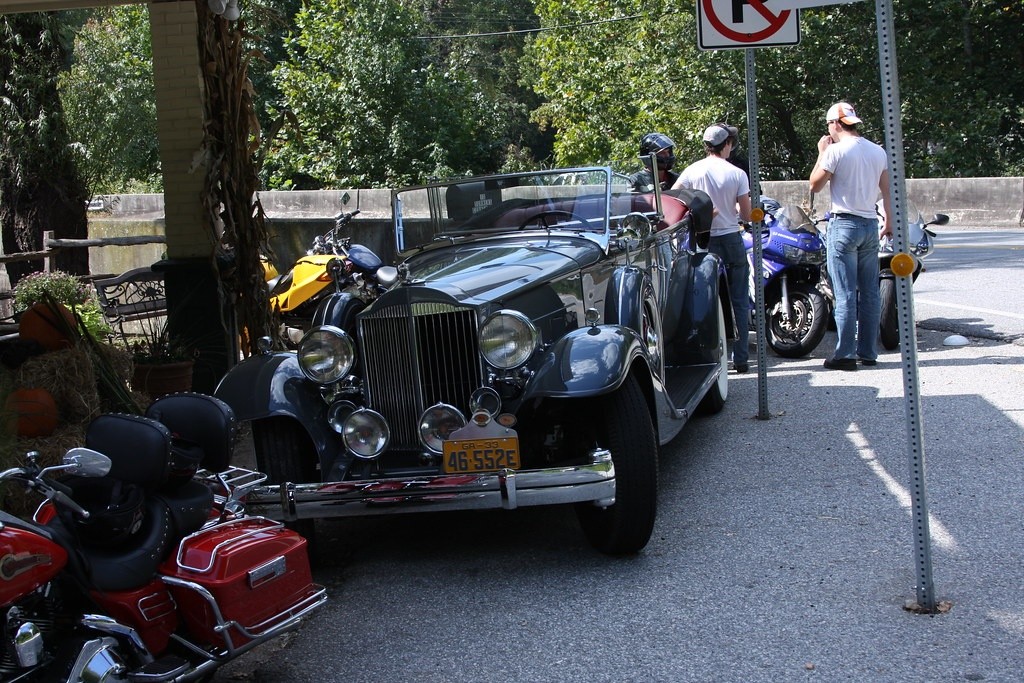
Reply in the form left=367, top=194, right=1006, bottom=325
left=51, top=473, right=146, bottom=552
left=640, top=133, right=677, bottom=170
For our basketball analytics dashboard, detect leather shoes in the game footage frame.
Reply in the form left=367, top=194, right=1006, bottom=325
left=857, top=358, right=876, bottom=366
left=824, top=359, right=857, bottom=370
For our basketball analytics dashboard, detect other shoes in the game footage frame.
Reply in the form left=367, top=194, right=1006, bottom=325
left=736, top=365, right=748, bottom=372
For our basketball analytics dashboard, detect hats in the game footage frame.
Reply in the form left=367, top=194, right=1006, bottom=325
left=825, top=101, right=863, bottom=125
left=703, top=123, right=739, bottom=146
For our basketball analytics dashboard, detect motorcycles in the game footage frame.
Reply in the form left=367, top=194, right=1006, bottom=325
left=732, top=182, right=951, bottom=361
left=252, top=189, right=381, bottom=352
left=0, top=391, right=331, bottom=683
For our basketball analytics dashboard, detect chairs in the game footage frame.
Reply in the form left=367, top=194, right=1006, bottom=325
left=94, top=266, right=170, bottom=358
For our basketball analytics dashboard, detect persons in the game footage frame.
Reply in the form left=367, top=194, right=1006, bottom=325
left=669, top=124, right=753, bottom=374
left=629, top=133, right=680, bottom=192
left=809, top=102, right=896, bottom=370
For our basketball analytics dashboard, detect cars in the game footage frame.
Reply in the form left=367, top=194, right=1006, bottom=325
left=252, top=133, right=741, bottom=559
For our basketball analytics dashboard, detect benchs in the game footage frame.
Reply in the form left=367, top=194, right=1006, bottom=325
left=457, top=187, right=712, bottom=237
